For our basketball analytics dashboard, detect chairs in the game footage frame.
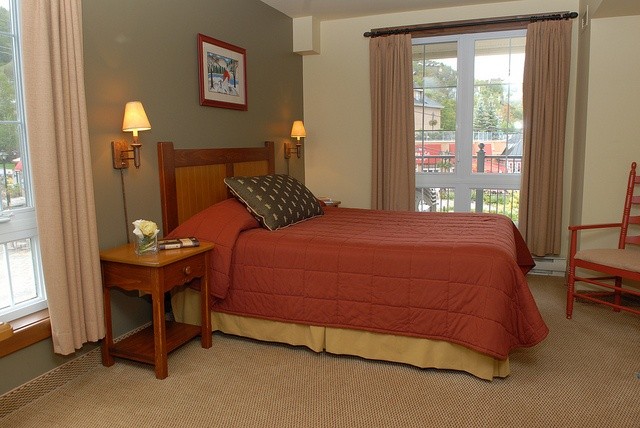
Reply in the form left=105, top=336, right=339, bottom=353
left=566, top=163, right=640, bottom=320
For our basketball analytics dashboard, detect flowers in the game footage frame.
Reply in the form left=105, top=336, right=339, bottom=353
left=132, top=219, right=161, bottom=252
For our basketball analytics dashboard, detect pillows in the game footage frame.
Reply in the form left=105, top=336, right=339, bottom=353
left=223, top=173, right=324, bottom=231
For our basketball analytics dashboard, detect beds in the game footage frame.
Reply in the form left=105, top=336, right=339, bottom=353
left=158, top=140, right=549, bottom=381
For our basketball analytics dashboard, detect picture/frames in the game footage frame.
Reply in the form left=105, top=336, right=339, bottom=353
left=197, top=32, right=248, bottom=110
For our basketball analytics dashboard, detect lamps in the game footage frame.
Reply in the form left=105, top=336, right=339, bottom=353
left=112, top=101, right=151, bottom=168
left=284, top=120, right=306, bottom=160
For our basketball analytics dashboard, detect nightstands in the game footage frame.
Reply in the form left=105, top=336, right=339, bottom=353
left=99, top=239, right=215, bottom=380
left=319, top=199, right=341, bottom=208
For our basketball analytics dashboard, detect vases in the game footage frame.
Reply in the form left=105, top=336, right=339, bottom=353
left=135, top=232, right=158, bottom=257
